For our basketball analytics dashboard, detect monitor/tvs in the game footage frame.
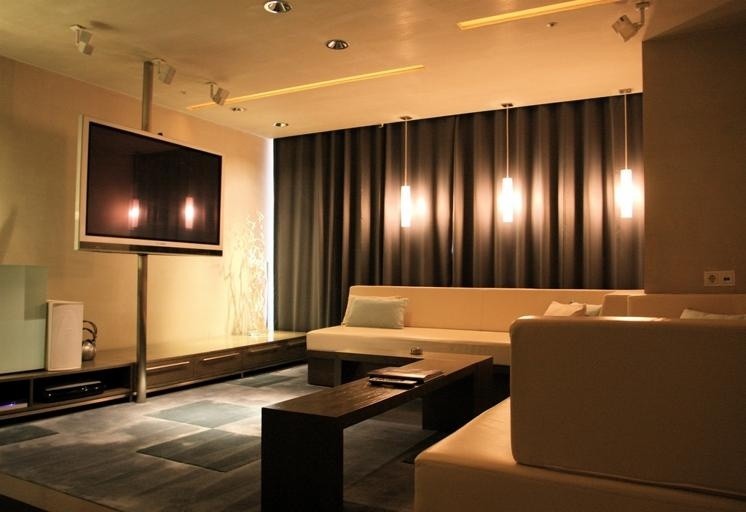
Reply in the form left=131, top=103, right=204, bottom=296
left=73, top=114, right=223, bottom=256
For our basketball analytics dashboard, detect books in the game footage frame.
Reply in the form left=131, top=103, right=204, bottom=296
left=365, top=365, right=444, bottom=387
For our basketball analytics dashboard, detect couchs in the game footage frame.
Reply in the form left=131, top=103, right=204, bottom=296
left=306, top=285, right=644, bottom=388
left=415, top=292, right=745, bottom=512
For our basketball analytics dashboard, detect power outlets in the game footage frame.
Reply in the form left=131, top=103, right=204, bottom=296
left=704, top=271, right=735, bottom=287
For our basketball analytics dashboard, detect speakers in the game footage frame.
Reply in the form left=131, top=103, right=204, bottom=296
left=46, top=299, right=84, bottom=371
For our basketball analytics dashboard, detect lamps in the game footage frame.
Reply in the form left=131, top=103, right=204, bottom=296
left=619, top=87, right=633, bottom=219
left=399, top=116, right=412, bottom=227
left=500, top=102, right=513, bottom=221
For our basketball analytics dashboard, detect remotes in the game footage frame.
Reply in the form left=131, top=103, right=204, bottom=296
left=368, top=377, right=418, bottom=388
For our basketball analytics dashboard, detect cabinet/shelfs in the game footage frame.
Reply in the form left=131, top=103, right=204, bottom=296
left=0, top=331, right=306, bottom=425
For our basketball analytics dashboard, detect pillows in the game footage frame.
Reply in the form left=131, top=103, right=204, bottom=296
left=680, top=308, right=746, bottom=323
left=543, top=300, right=602, bottom=317
left=342, top=294, right=408, bottom=329
